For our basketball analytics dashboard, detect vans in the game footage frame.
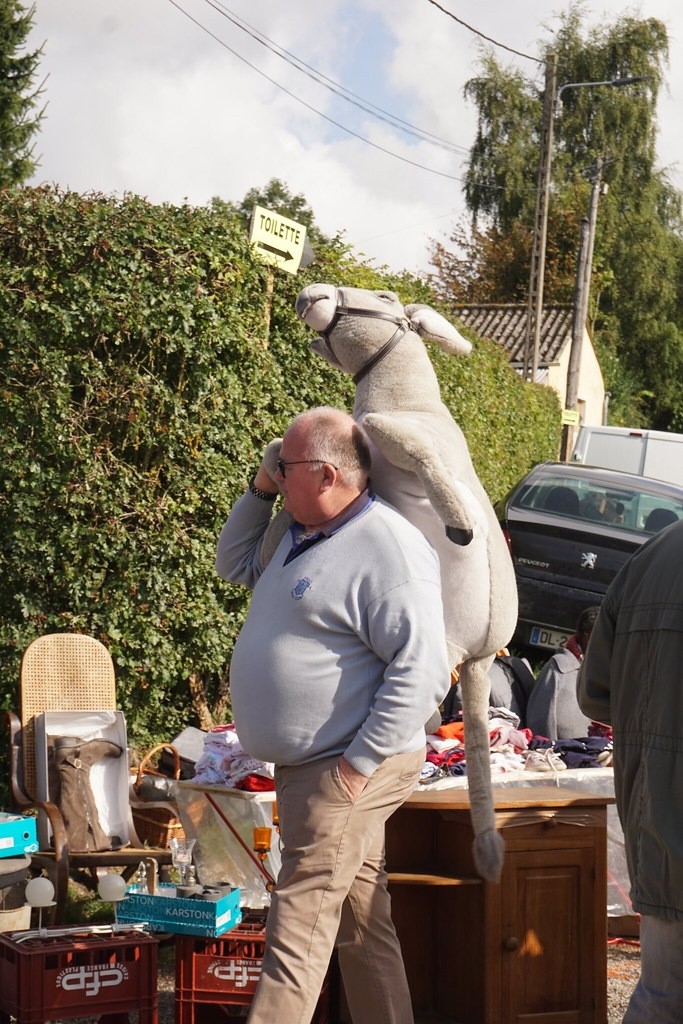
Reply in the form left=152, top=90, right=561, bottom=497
left=562, top=424, right=683, bottom=537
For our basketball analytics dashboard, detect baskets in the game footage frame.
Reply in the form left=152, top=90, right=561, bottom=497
left=132, top=743, right=206, bottom=851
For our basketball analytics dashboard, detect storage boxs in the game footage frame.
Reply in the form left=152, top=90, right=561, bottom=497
left=158, top=726, right=207, bottom=780
left=171, top=923, right=325, bottom=1024
left=32, top=708, right=132, bottom=852
left=117, top=882, right=244, bottom=937
left=0, top=812, right=38, bottom=858
left=129, top=743, right=205, bottom=849
left=0, top=930, right=160, bottom=1024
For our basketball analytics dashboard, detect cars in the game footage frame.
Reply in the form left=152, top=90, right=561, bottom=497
left=495, top=458, right=683, bottom=654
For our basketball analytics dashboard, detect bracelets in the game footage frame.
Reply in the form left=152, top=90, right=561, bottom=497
left=249, top=477, right=276, bottom=501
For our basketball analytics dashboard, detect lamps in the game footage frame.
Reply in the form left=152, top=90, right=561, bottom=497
left=97, top=874, right=130, bottom=925
left=24, top=878, right=57, bottom=930
left=254, top=803, right=287, bottom=893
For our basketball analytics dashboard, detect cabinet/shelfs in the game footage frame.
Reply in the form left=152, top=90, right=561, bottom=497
left=273, top=789, right=617, bottom=1024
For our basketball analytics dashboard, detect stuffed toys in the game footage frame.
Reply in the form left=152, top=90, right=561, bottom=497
left=263, top=283, right=520, bottom=885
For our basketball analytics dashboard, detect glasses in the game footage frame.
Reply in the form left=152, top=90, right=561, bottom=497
left=276, top=456, right=337, bottom=479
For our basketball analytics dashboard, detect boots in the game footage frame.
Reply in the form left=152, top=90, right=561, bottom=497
left=50, top=735, right=125, bottom=852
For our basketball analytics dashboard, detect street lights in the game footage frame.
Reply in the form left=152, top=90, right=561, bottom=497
left=528, top=76, right=644, bottom=382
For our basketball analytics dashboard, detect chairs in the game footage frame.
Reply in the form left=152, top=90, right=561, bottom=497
left=0, top=633, right=159, bottom=930
left=543, top=484, right=582, bottom=514
left=641, top=508, right=678, bottom=533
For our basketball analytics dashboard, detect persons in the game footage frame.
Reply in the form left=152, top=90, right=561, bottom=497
left=444, top=652, right=535, bottom=730
left=576, top=516, right=683, bottom=1024
left=524, top=606, right=600, bottom=742
left=215, top=406, right=449, bottom=1024
